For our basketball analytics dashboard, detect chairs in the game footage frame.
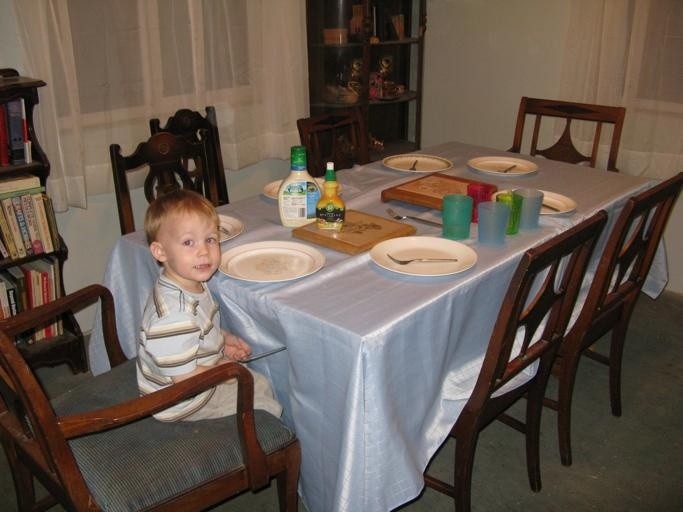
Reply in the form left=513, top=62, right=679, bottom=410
left=520, top=172, right=683, bottom=466
left=150, top=106, right=229, bottom=206
left=507, top=95, right=626, bottom=173
left=0, top=282, right=302, bottom=512
left=297, top=105, right=370, bottom=178
left=423, top=209, right=608, bottom=511
left=109, top=128, right=226, bottom=235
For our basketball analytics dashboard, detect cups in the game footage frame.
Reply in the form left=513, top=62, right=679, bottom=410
left=477, top=201, right=510, bottom=249
left=513, top=187, right=544, bottom=231
left=466, top=183, right=498, bottom=224
left=495, top=194, right=523, bottom=236
left=442, top=194, right=475, bottom=241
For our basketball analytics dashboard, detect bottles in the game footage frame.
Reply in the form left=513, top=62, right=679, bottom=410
left=277, top=144, right=323, bottom=230
left=316, top=161, right=345, bottom=231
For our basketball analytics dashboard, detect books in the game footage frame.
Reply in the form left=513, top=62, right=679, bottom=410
left=0, top=100, right=66, bottom=346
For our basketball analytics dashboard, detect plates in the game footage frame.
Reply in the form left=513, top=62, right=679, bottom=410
left=466, top=155, right=540, bottom=178
left=368, top=236, right=478, bottom=280
left=490, top=187, right=577, bottom=217
left=262, top=177, right=343, bottom=200
left=381, top=154, right=454, bottom=174
left=217, top=239, right=327, bottom=285
left=217, top=214, right=246, bottom=244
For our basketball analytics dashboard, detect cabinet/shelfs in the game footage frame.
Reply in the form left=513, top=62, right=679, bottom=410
left=0, top=67, right=88, bottom=369
left=306, top=0, right=427, bottom=165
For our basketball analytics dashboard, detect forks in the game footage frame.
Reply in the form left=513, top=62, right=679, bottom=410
left=385, top=253, right=460, bottom=266
left=384, top=207, right=443, bottom=231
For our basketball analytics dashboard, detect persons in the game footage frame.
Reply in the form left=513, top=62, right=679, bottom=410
left=134, top=186, right=282, bottom=424
